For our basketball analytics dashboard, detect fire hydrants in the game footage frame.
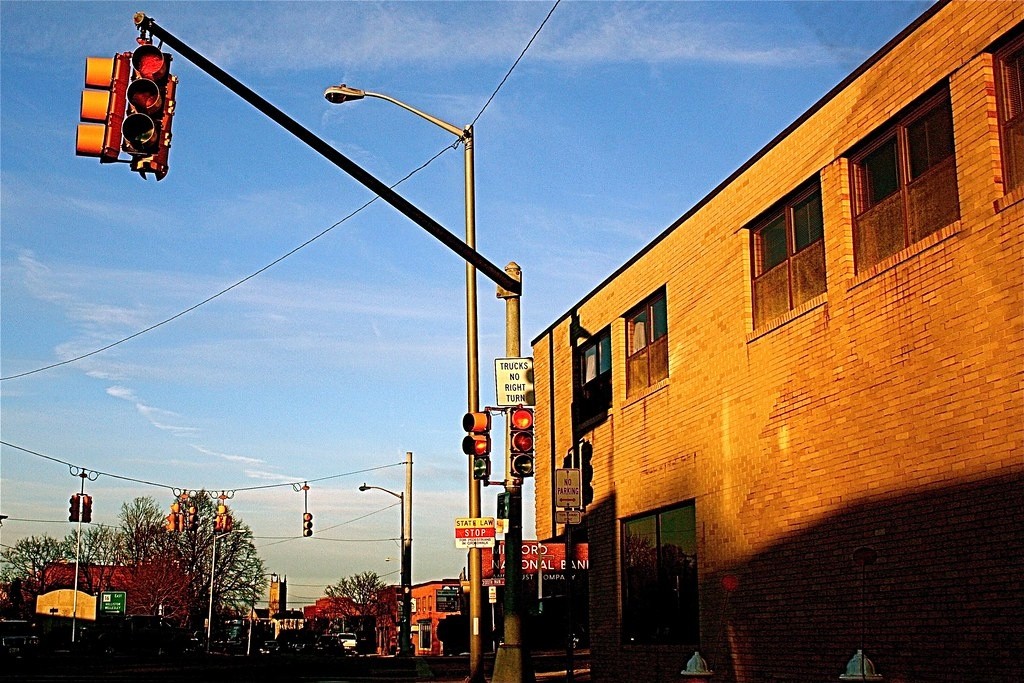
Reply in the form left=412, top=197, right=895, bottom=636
left=680, top=651, right=715, bottom=683
left=837, top=648, right=883, bottom=683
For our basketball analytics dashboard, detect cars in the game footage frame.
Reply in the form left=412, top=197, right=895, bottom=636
left=0, top=614, right=361, bottom=661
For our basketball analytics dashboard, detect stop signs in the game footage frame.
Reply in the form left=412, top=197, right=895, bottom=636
left=454, top=517, right=496, bottom=548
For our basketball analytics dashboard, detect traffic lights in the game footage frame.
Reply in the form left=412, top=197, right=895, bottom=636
left=121, top=43, right=174, bottom=156
left=461, top=409, right=491, bottom=457
left=188, top=505, right=198, bottom=531
left=302, top=512, right=313, bottom=537
left=222, top=513, right=232, bottom=533
left=81, top=495, right=92, bottom=523
left=507, top=404, right=533, bottom=476
left=67, top=494, right=78, bottom=522
left=472, top=457, right=487, bottom=478
left=165, top=514, right=175, bottom=531
left=74, top=50, right=130, bottom=163
left=211, top=512, right=222, bottom=533
left=176, top=512, right=184, bottom=532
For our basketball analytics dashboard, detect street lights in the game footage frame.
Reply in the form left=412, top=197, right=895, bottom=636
left=206, top=528, right=246, bottom=639
left=324, top=82, right=485, bottom=683
left=358, top=483, right=403, bottom=586
left=245, top=571, right=277, bottom=656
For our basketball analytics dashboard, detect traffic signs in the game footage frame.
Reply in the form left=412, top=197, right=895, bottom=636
left=100, top=589, right=127, bottom=617
left=495, top=356, right=535, bottom=406
left=556, top=509, right=582, bottom=525
left=555, top=468, right=581, bottom=508
